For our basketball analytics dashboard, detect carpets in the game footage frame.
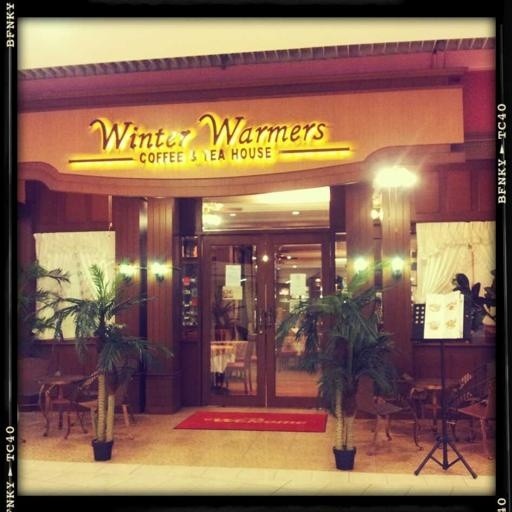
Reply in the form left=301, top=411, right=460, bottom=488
left=175, top=411, right=327, bottom=432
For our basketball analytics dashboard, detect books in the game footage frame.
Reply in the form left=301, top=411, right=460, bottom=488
left=423, top=289, right=464, bottom=341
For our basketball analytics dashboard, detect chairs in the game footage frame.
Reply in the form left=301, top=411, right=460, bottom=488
left=73, top=364, right=137, bottom=436
left=345, top=362, right=496, bottom=460
left=225, top=339, right=254, bottom=394
left=15, top=353, right=71, bottom=443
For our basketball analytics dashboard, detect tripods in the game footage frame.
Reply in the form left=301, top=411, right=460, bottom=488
left=412, top=341, right=480, bottom=482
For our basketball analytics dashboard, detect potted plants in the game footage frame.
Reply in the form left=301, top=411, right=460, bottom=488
left=274, top=259, right=403, bottom=469
left=34, top=262, right=175, bottom=460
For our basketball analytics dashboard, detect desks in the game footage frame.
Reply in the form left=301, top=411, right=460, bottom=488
left=34, top=375, right=85, bottom=437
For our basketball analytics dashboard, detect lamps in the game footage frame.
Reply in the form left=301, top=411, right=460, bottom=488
left=153, top=262, right=166, bottom=280
left=119, top=262, right=132, bottom=283
left=392, top=256, right=403, bottom=279
